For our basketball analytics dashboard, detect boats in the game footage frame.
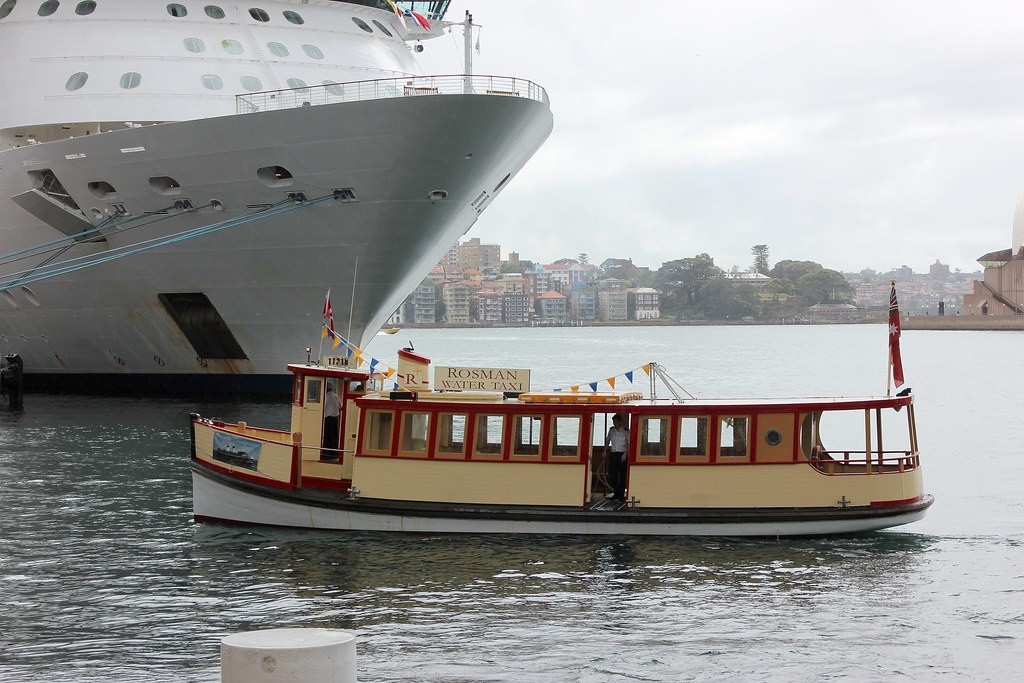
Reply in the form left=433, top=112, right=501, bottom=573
left=189, top=280, right=936, bottom=540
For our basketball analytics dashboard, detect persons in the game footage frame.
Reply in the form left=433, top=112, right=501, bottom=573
left=601, top=415, right=630, bottom=502
left=323, top=383, right=343, bottom=454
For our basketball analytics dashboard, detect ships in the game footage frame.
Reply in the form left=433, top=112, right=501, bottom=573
left=0, top=2, right=553, bottom=396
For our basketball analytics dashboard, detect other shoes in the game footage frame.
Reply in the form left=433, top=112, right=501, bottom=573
left=610, top=495, right=618, bottom=499
left=619, top=498, right=625, bottom=502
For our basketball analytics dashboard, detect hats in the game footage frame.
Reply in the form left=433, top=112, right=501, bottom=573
left=327, top=383, right=332, bottom=389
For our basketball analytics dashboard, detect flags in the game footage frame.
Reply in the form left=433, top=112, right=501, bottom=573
left=888, top=287, right=904, bottom=387
left=323, top=293, right=335, bottom=340
left=387, top=0, right=431, bottom=32
